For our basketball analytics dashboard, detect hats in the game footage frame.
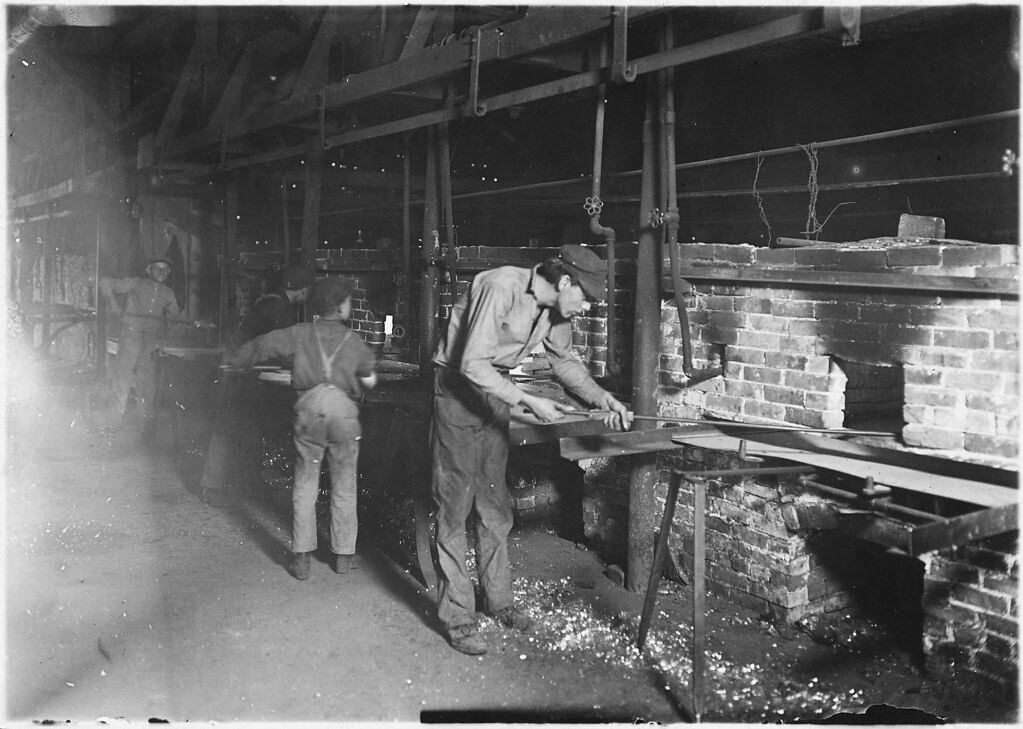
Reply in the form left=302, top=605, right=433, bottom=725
left=281, top=266, right=314, bottom=289
left=149, top=257, right=174, bottom=268
left=557, top=244, right=607, bottom=302
left=310, top=277, right=351, bottom=310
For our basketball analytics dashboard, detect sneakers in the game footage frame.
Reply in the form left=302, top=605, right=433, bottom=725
left=482, top=606, right=535, bottom=634
left=444, top=623, right=488, bottom=654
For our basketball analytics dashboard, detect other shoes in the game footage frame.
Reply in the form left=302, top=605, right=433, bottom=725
left=288, top=552, right=310, bottom=580
left=198, top=486, right=233, bottom=509
left=330, top=553, right=352, bottom=573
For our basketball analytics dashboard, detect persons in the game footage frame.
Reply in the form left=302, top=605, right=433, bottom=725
left=428, top=244, right=632, bottom=655
left=200, top=273, right=316, bottom=508
left=228, top=275, right=378, bottom=580
left=100, top=255, right=185, bottom=451
